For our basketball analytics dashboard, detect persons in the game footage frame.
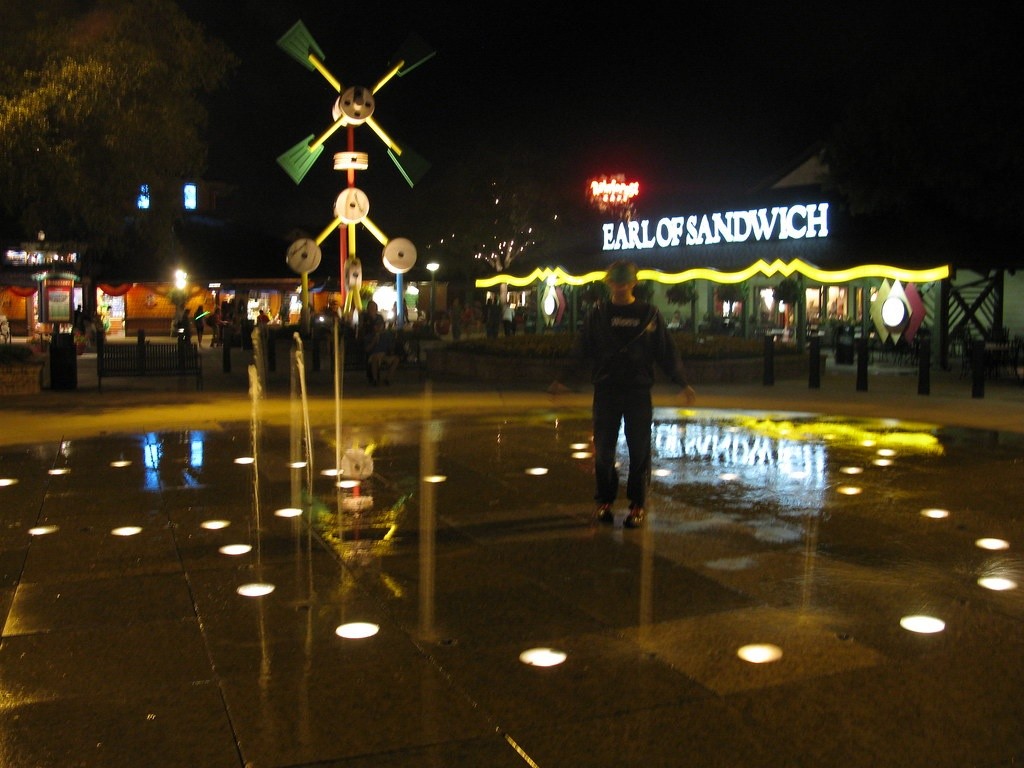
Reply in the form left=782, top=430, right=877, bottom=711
left=177, top=309, right=192, bottom=341
left=364, top=301, right=385, bottom=329
left=450, top=298, right=516, bottom=338
left=208, top=309, right=222, bottom=345
left=71, top=305, right=83, bottom=335
left=366, top=321, right=398, bottom=383
left=257, top=310, right=270, bottom=324
left=549, top=260, right=697, bottom=523
left=194, top=306, right=205, bottom=345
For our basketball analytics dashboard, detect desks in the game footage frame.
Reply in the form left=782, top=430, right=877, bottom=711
left=985, top=342, right=1014, bottom=383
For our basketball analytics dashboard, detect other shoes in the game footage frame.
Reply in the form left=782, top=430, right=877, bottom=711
left=626, top=504, right=645, bottom=527
left=597, top=503, right=615, bottom=523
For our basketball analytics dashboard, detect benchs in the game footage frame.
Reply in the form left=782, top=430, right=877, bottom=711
left=96, top=329, right=204, bottom=392
left=327, top=328, right=429, bottom=386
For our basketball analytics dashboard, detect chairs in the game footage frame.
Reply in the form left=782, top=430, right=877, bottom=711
left=870, top=326, right=1024, bottom=384
